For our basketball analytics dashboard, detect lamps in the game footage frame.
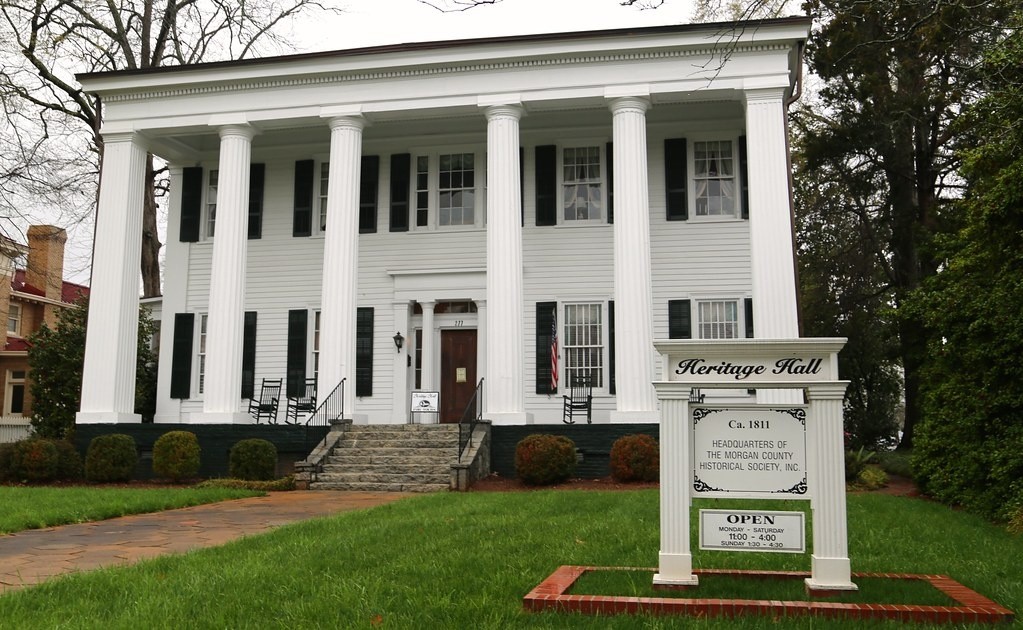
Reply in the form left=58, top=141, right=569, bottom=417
left=393, top=331, right=403, bottom=353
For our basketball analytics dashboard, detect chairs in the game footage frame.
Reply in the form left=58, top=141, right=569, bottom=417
left=563, top=375, right=594, bottom=424
left=285, top=373, right=318, bottom=426
left=248, top=378, right=283, bottom=424
